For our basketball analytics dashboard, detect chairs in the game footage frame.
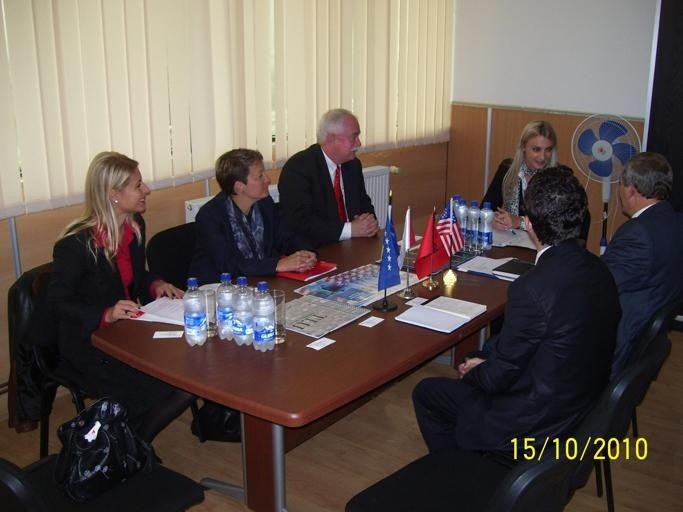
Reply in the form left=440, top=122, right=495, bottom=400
left=8, top=262, right=199, bottom=463
left=346, top=444, right=585, bottom=512
left=147, top=221, right=197, bottom=292
left=490, top=331, right=672, bottom=512
left=620, top=291, right=683, bottom=438
left=0, top=448, right=205, bottom=512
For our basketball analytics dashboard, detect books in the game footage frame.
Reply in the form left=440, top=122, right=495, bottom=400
left=276, top=255, right=338, bottom=281
left=395, top=295, right=487, bottom=335
left=457, top=255, right=516, bottom=282
left=492, top=260, right=535, bottom=279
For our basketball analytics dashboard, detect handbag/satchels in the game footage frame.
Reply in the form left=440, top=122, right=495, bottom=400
left=52, top=397, right=157, bottom=505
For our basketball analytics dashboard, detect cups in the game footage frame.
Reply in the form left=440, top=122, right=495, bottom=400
left=196, top=289, right=216, bottom=338
left=262, top=290, right=286, bottom=346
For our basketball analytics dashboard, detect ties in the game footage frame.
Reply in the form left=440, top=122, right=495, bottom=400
left=334, top=165, right=347, bottom=224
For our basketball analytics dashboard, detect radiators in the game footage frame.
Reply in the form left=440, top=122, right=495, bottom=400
left=184, top=165, right=400, bottom=230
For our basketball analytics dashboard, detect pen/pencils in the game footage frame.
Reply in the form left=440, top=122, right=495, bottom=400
left=494, top=217, right=517, bottom=235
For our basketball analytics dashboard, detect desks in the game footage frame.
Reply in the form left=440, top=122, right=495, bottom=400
left=92, top=212, right=540, bottom=512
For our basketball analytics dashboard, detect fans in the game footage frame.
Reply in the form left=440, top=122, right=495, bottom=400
left=570, top=113, right=641, bottom=259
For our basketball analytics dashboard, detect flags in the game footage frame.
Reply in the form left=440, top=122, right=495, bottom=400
left=397, top=208, right=416, bottom=270
left=378, top=205, right=401, bottom=291
left=416, top=212, right=450, bottom=280
left=435, top=200, right=464, bottom=257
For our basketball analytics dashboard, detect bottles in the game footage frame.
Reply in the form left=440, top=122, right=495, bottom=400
left=216, top=273, right=275, bottom=353
left=182, top=277, right=207, bottom=347
left=449, top=194, right=492, bottom=251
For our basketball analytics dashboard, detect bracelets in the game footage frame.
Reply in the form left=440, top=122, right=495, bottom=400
left=520, top=215, right=524, bottom=231
left=108, top=306, right=114, bottom=321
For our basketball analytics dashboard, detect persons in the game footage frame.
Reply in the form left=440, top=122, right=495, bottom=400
left=52, top=151, right=198, bottom=443
left=600, top=152, right=682, bottom=382
left=479, top=120, right=560, bottom=231
left=186, top=147, right=317, bottom=285
left=277, top=108, right=380, bottom=248
left=412, top=166, right=622, bottom=469
